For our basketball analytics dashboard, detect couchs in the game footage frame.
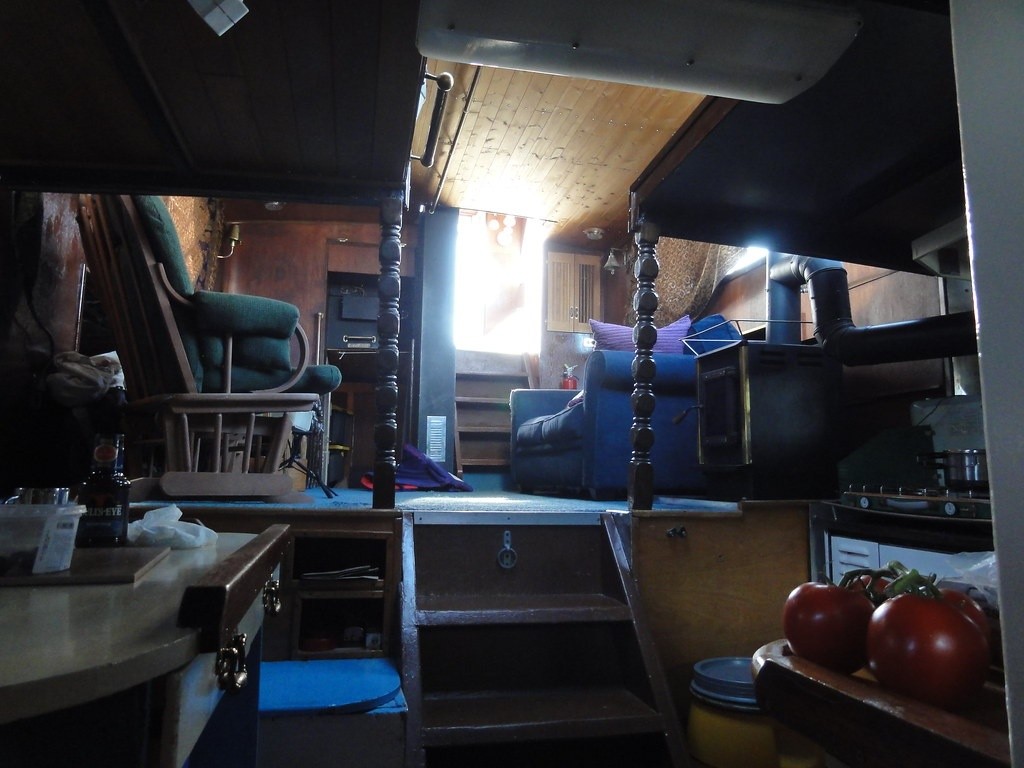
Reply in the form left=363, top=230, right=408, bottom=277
left=509, top=349, right=710, bottom=499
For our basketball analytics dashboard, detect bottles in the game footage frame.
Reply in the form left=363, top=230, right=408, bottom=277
left=71, top=434, right=129, bottom=548
left=117, top=434, right=132, bottom=498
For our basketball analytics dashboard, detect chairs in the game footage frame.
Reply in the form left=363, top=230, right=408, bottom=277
left=74, top=192, right=342, bottom=500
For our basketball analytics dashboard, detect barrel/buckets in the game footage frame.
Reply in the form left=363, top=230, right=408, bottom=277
left=686, top=658, right=827, bottom=768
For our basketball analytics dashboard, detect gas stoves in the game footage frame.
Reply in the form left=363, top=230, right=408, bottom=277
left=842, top=483, right=991, bottom=520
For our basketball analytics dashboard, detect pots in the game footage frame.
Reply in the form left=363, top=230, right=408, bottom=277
left=917, top=447, right=989, bottom=490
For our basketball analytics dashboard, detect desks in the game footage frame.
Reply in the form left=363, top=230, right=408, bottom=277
left=1, top=522, right=291, bottom=768
left=628, top=0, right=972, bottom=509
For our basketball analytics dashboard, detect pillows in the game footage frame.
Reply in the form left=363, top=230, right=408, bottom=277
left=567, top=314, right=693, bottom=407
left=684, top=313, right=739, bottom=357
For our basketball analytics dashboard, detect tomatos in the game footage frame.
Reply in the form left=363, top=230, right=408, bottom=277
left=781, top=562, right=990, bottom=701
